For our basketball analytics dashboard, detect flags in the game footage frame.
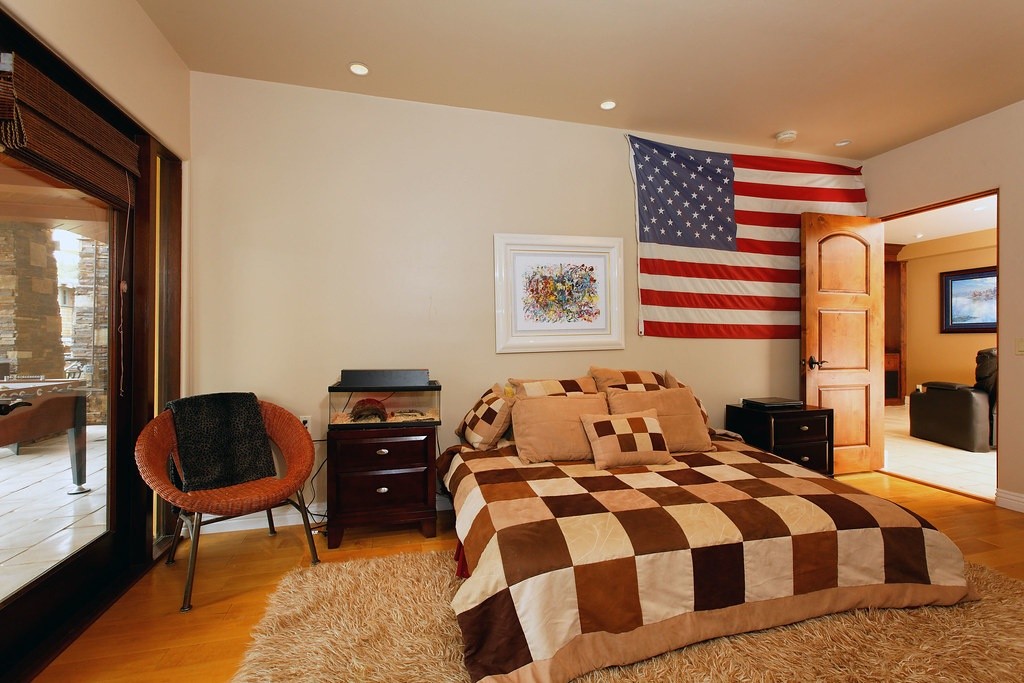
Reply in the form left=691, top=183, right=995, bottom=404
left=624, top=133, right=868, bottom=340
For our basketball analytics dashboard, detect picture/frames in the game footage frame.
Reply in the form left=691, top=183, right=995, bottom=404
left=938, top=266, right=998, bottom=333
left=493, top=233, right=627, bottom=355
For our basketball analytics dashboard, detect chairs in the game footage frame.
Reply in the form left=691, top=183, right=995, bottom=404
left=133, top=394, right=321, bottom=609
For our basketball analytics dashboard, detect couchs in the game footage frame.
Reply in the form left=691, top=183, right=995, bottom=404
left=910, top=347, right=997, bottom=454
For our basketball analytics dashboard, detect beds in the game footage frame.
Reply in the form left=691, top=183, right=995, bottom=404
left=444, top=430, right=979, bottom=682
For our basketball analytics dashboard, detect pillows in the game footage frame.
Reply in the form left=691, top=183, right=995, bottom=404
left=509, top=374, right=598, bottom=398
left=590, top=366, right=667, bottom=394
left=607, top=385, right=716, bottom=453
left=453, top=384, right=514, bottom=451
left=512, top=393, right=607, bottom=464
left=579, top=410, right=675, bottom=471
left=663, top=370, right=709, bottom=427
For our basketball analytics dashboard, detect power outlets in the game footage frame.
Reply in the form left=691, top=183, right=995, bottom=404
left=916, top=384, right=922, bottom=393
left=298, top=416, right=312, bottom=436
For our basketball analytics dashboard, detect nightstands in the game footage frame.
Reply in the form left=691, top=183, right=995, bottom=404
left=326, top=426, right=440, bottom=549
left=725, top=401, right=836, bottom=483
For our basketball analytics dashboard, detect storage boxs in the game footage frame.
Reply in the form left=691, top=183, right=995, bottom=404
left=327, top=381, right=442, bottom=424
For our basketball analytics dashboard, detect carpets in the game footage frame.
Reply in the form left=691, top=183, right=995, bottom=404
left=233, top=551, right=1024, bottom=683
